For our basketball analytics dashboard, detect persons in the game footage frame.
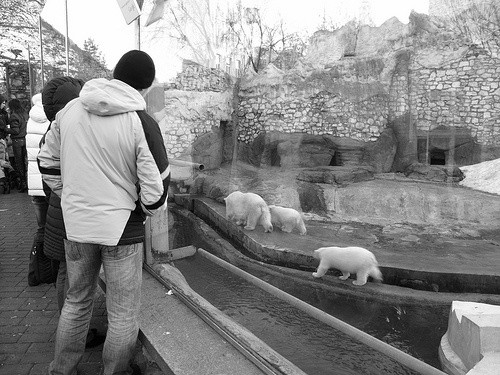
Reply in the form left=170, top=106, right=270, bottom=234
left=5, top=99, right=28, bottom=192
left=25, top=93, right=60, bottom=287
left=0, top=94, right=9, bottom=139
left=36, top=50, right=170, bottom=375
left=42, top=76, right=100, bottom=347
left=0, top=138, right=14, bottom=194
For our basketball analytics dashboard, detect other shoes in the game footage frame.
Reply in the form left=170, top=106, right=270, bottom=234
left=85, top=329, right=107, bottom=348
left=0, top=177, right=29, bottom=194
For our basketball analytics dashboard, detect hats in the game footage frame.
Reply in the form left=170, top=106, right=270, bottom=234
left=115, top=49, right=156, bottom=89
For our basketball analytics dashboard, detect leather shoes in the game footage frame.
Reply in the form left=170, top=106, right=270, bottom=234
left=128, top=364, right=142, bottom=375
